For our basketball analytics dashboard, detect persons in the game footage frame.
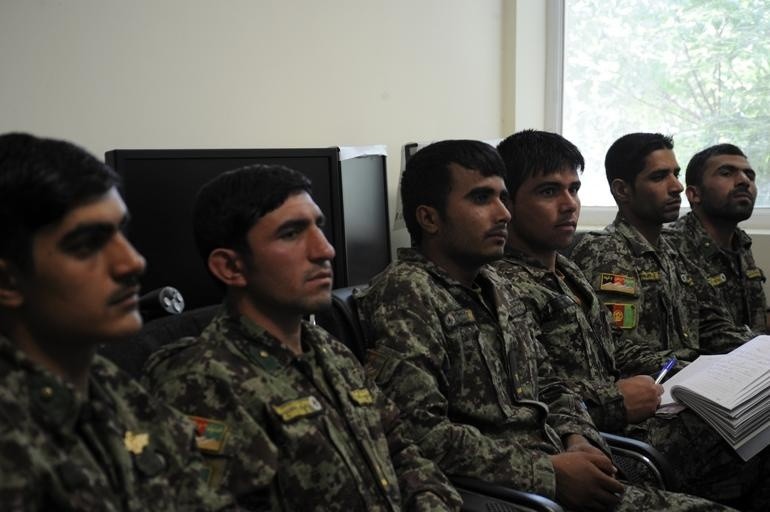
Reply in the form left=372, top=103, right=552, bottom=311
left=352, top=139, right=734, bottom=512
left=136, top=161, right=463, bottom=511
left=669, top=142, right=769, bottom=335
left=557, top=133, right=750, bottom=386
left=0, top=131, right=229, bottom=512
left=492, top=127, right=768, bottom=501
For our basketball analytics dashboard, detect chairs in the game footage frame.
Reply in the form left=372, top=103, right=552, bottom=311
left=311, top=283, right=673, bottom=511
left=101, top=300, right=570, bottom=512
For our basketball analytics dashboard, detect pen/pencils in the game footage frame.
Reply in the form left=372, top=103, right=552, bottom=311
left=654, top=359, right=677, bottom=385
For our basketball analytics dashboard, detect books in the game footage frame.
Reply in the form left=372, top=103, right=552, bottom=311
left=657, top=333, right=769, bottom=462
